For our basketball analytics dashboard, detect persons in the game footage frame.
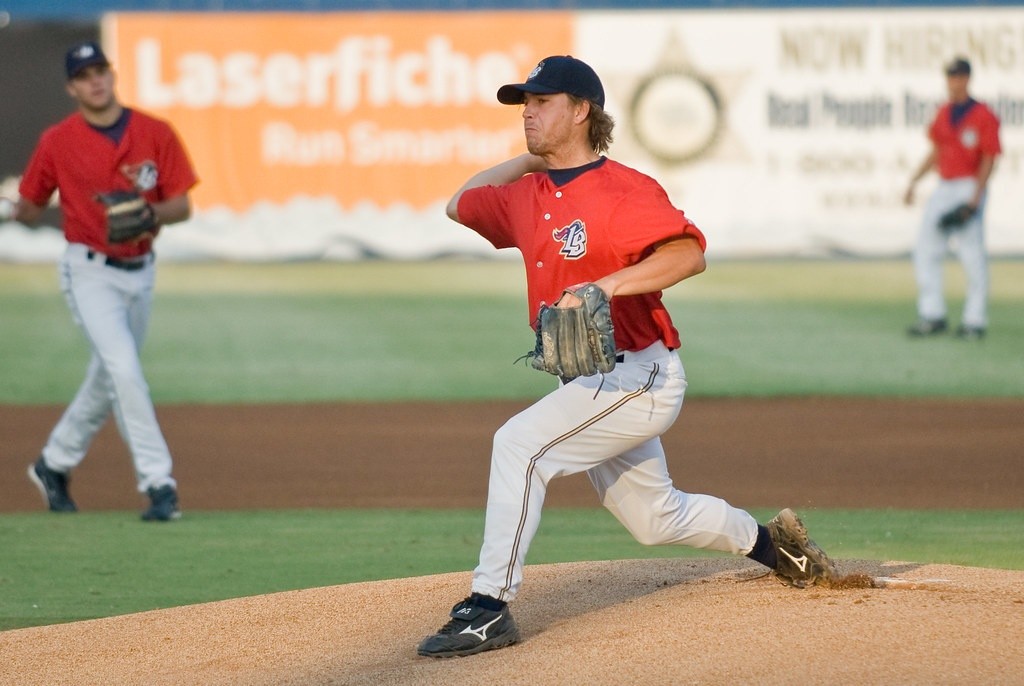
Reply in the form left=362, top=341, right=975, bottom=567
left=902, top=57, right=1000, bottom=339
left=0, top=41, right=197, bottom=519
left=416, top=54, right=840, bottom=658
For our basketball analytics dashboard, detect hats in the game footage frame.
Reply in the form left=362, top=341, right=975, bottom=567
left=65, top=42, right=108, bottom=79
left=946, top=60, right=971, bottom=75
left=497, top=55, right=605, bottom=108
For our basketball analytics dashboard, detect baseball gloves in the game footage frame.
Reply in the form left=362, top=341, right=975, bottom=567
left=937, top=205, right=976, bottom=228
left=530, top=281, right=617, bottom=379
left=92, top=186, right=158, bottom=244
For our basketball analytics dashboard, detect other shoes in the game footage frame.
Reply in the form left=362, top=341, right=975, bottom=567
left=907, top=319, right=947, bottom=335
left=957, top=326, right=985, bottom=339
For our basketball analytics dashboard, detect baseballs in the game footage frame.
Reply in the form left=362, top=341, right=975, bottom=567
left=0, top=198, right=16, bottom=220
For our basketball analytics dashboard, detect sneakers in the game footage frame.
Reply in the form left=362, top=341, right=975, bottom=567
left=143, top=485, right=182, bottom=520
left=417, top=596, right=521, bottom=659
left=27, top=456, right=76, bottom=513
left=736, top=508, right=840, bottom=590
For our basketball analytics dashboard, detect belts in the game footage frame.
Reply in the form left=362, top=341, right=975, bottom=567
left=88, top=252, right=144, bottom=270
left=616, top=345, right=674, bottom=362
